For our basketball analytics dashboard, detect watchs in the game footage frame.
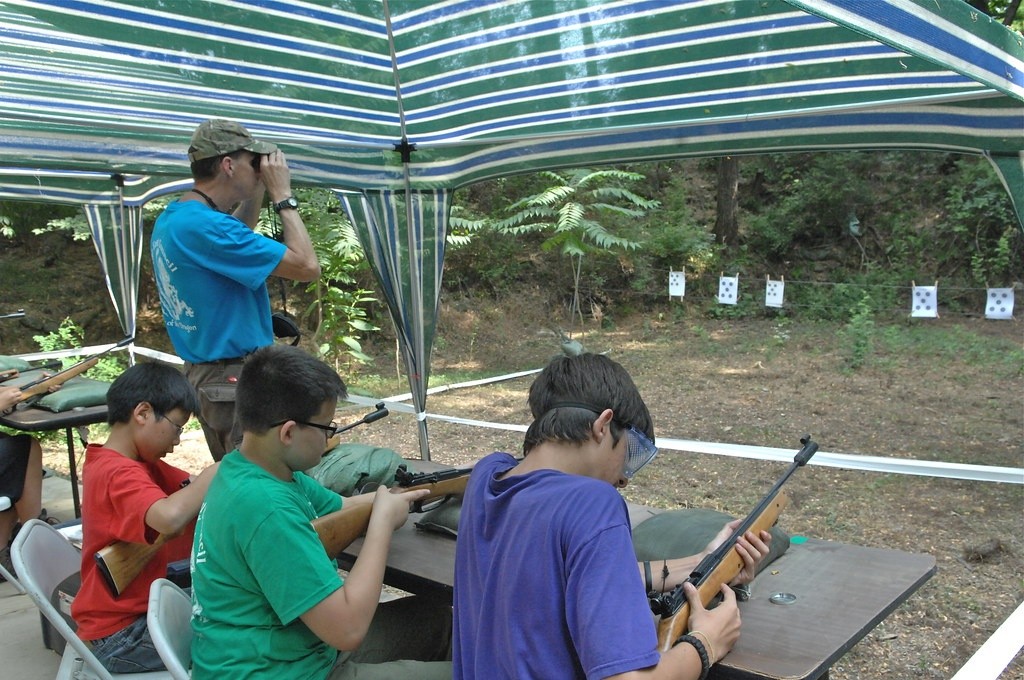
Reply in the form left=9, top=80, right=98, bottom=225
left=274, top=197, right=297, bottom=215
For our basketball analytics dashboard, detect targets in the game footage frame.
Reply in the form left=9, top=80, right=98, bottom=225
left=718, top=276, right=738, bottom=305
left=765, top=280, right=784, bottom=308
left=668, top=271, right=686, bottom=297
left=911, top=285, right=938, bottom=318
left=984, top=288, right=1015, bottom=320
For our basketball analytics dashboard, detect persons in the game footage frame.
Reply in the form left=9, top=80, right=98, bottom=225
left=184, top=346, right=455, bottom=679
left=150, top=119, right=321, bottom=462
left=453, top=354, right=771, bottom=680
left=71, top=361, right=222, bottom=673
left=0, top=372, right=63, bottom=584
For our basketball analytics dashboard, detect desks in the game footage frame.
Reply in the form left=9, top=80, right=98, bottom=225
left=0, top=367, right=108, bottom=519
left=335, top=459, right=936, bottom=680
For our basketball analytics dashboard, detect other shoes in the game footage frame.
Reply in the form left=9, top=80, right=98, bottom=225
left=0, top=547, right=18, bottom=583
left=8, top=509, right=58, bottom=546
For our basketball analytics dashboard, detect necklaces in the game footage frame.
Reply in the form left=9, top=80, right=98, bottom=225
left=191, top=187, right=220, bottom=212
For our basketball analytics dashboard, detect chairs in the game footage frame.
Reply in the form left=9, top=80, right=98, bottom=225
left=0, top=496, right=26, bottom=596
left=11, top=518, right=191, bottom=680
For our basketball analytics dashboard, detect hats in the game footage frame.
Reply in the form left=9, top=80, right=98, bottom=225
left=188, top=119, right=277, bottom=163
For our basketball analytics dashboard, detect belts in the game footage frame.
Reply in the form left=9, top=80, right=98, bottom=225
left=199, top=357, right=246, bottom=365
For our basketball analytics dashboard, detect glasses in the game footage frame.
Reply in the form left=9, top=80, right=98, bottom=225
left=544, top=401, right=658, bottom=480
left=164, top=415, right=183, bottom=437
left=271, top=419, right=338, bottom=438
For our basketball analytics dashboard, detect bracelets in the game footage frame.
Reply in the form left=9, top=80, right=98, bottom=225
left=643, top=560, right=653, bottom=598
left=673, top=635, right=709, bottom=680
left=662, top=559, right=669, bottom=596
left=686, top=631, right=714, bottom=670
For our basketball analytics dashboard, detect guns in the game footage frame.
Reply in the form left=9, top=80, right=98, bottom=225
left=7, top=333, right=138, bottom=406
left=309, top=464, right=473, bottom=563
left=93, top=400, right=390, bottom=598
left=0, top=360, right=63, bottom=383
left=647, top=433, right=821, bottom=653
